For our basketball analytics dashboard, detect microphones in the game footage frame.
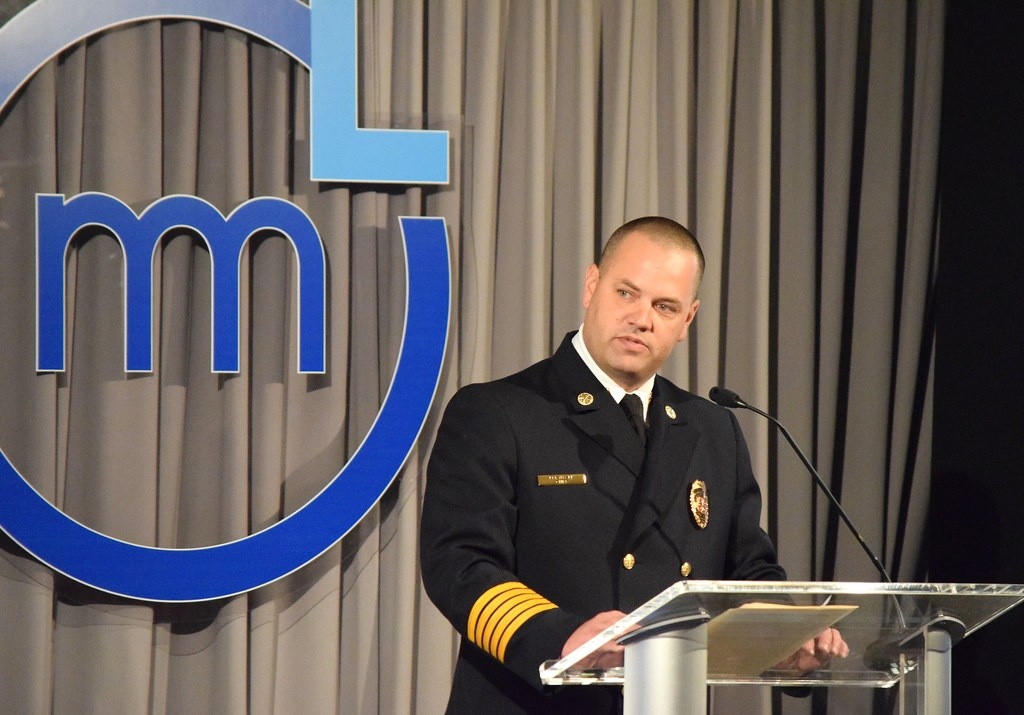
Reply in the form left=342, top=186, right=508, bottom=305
left=708, top=385, right=891, bottom=582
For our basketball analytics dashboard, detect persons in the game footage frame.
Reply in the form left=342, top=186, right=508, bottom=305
left=417, top=215, right=849, bottom=715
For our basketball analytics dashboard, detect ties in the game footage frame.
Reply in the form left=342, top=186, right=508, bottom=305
left=619, top=395, right=648, bottom=452
